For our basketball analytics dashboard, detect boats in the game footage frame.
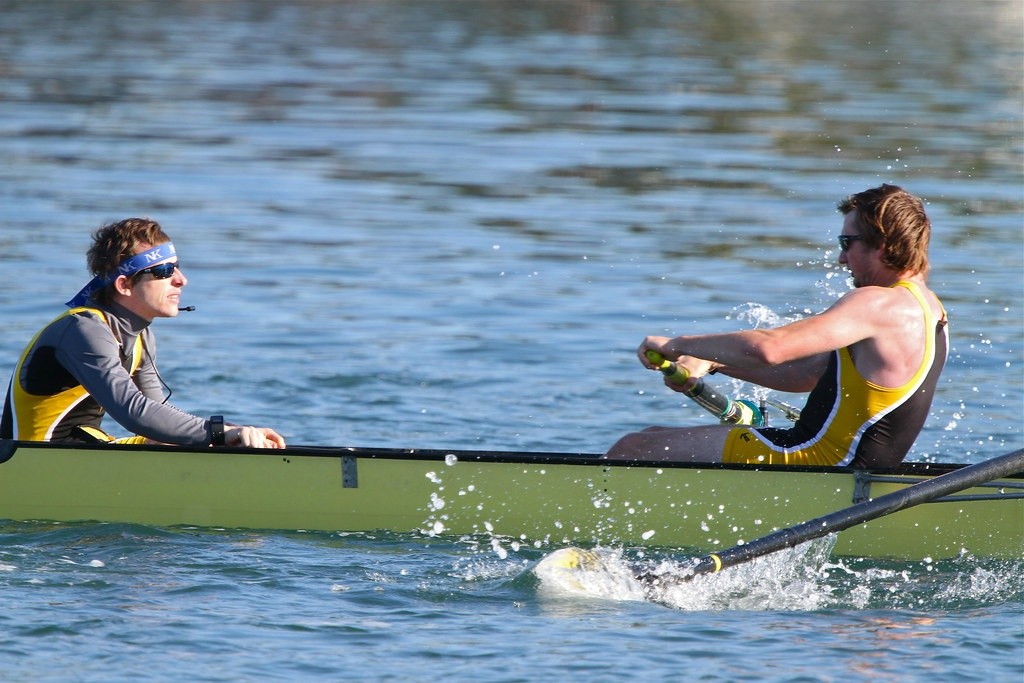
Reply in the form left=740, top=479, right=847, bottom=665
left=0, top=439, right=1024, bottom=565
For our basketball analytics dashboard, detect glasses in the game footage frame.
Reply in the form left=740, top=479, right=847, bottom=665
left=838, top=234, right=886, bottom=251
left=128, top=260, right=179, bottom=279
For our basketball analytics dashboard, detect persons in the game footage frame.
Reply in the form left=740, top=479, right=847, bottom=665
left=602, top=183, right=950, bottom=465
left=0, top=217, right=289, bottom=450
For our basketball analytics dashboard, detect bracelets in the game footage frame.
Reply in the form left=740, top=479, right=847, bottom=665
left=210, top=414, right=226, bottom=448
